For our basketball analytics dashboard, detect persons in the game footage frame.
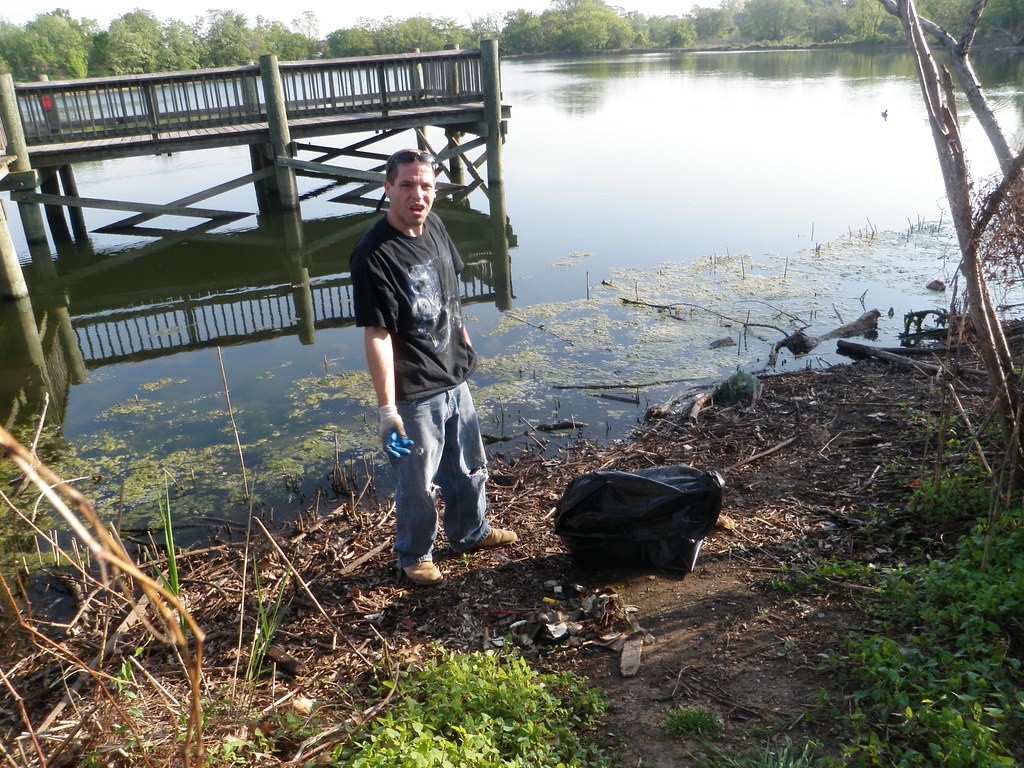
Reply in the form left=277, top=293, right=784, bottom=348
left=350, top=148, right=517, bottom=585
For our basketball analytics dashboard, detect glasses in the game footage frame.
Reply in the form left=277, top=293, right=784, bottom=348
left=387, top=149, right=437, bottom=179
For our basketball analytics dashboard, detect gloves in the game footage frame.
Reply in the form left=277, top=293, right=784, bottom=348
left=377, top=405, right=415, bottom=458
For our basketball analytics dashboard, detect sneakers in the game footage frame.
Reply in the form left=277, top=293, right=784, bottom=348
left=399, top=561, right=444, bottom=584
left=475, top=527, right=517, bottom=548
